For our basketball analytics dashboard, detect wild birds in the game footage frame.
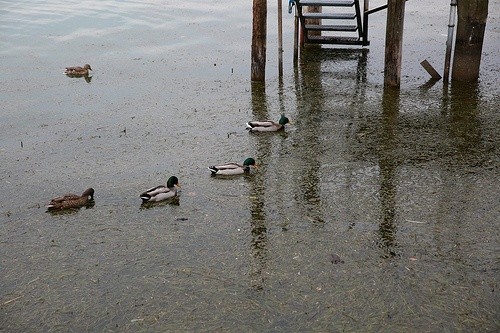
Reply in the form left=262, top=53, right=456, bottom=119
left=207, top=157, right=259, bottom=176
left=139, top=175, right=182, bottom=203
left=44, top=188, right=94, bottom=210
left=245, top=115, right=292, bottom=134
left=64, top=64, right=93, bottom=75
